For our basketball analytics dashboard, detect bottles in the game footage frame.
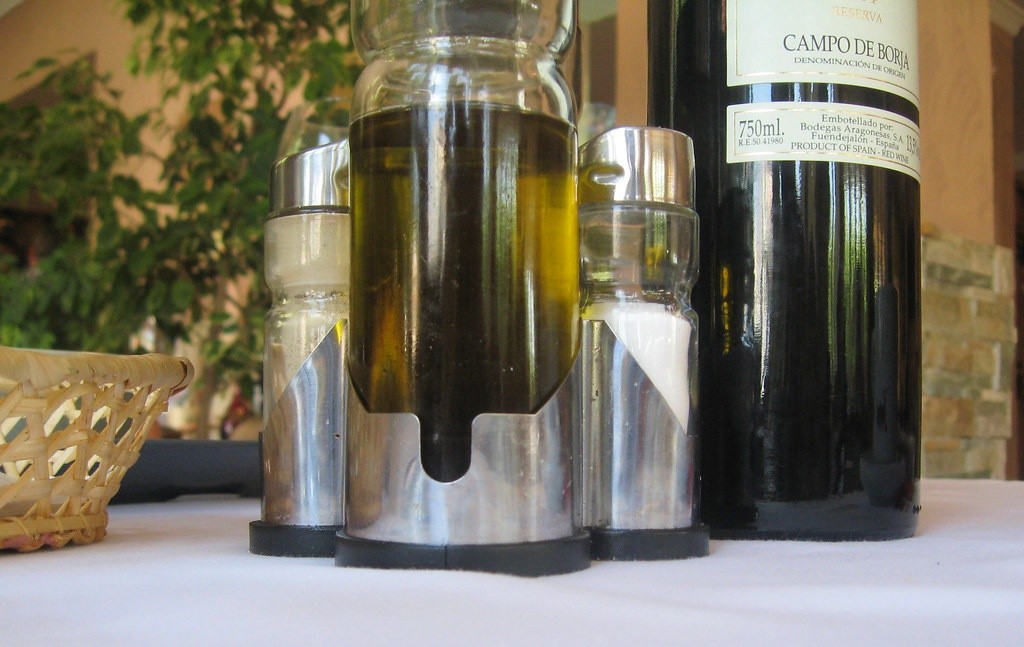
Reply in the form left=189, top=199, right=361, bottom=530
left=344, top=1, right=580, bottom=546
left=573, top=128, right=699, bottom=533
left=260, top=137, right=350, bottom=526
left=645, top=0, right=923, bottom=541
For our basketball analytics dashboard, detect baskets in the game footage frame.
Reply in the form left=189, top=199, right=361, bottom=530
left=0, top=345, right=196, bottom=555
